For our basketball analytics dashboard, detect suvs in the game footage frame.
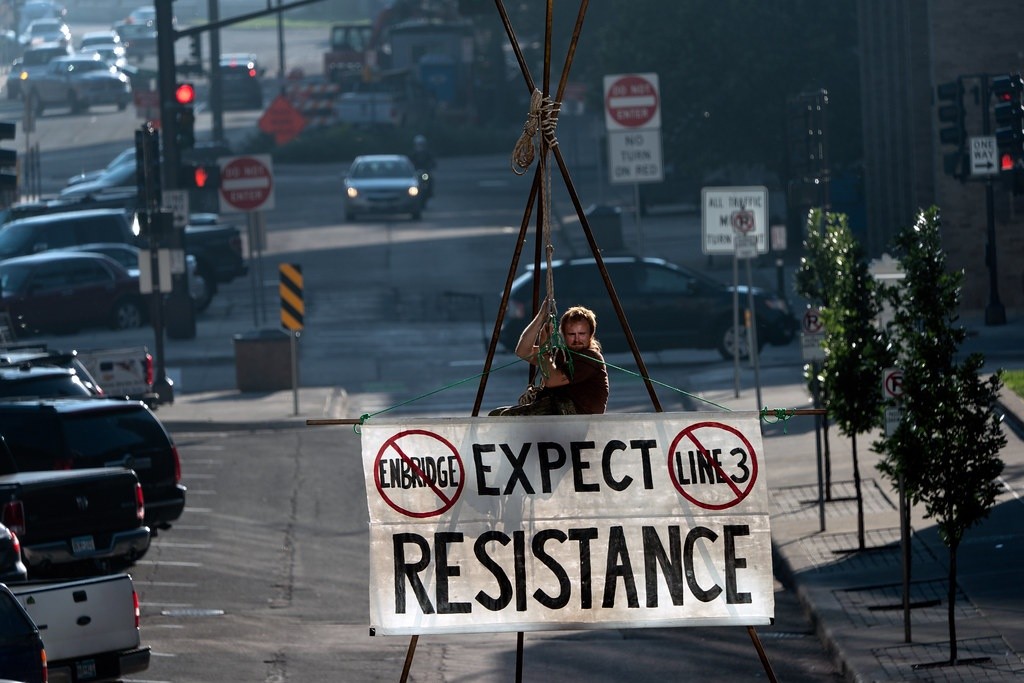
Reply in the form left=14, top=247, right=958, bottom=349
left=491, top=254, right=797, bottom=363
left=0, top=339, right=187, bottom=683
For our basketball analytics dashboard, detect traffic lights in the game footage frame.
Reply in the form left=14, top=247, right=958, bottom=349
left=171, top=81, right=194, bottom=153
left=194, top=162, right=222, bottom=192
left=935, top=78, right=965, bottom=176
left=189, top=33, right=201, bottom=59
left=990, top=73, right=1024, bottom=178
left=0, top=122, right=17, bottom=189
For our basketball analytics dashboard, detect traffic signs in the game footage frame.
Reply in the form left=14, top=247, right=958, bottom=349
left=969, top=137, right=1000, bottom=177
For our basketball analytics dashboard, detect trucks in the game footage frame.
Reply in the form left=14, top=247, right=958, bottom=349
left=271, top=1, right=512, bottom=166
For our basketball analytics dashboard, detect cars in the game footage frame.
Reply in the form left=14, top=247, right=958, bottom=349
left=0, top=0, right=177, bottom=117
left=343, top=154, right=420, bottom=225
left=0, top=147, right=242, bottom=342
left=216, top=53, right=265, bottom=111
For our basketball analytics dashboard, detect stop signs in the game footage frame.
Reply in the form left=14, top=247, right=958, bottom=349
left=215, top=154, right=276, bottom=216
left=603, top=74, right=662, bottom=130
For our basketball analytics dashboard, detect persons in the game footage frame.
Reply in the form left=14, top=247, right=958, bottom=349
left=449, top=306, right=609, bottom=539
left=411, top=136, right=432, bottom=171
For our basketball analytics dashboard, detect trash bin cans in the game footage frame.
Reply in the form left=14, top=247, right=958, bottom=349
left=183, top=224, right=242, bottom=283
left=585, top=203, right=622, bottom=252
left=232, top=329, right=292, bottom=395
